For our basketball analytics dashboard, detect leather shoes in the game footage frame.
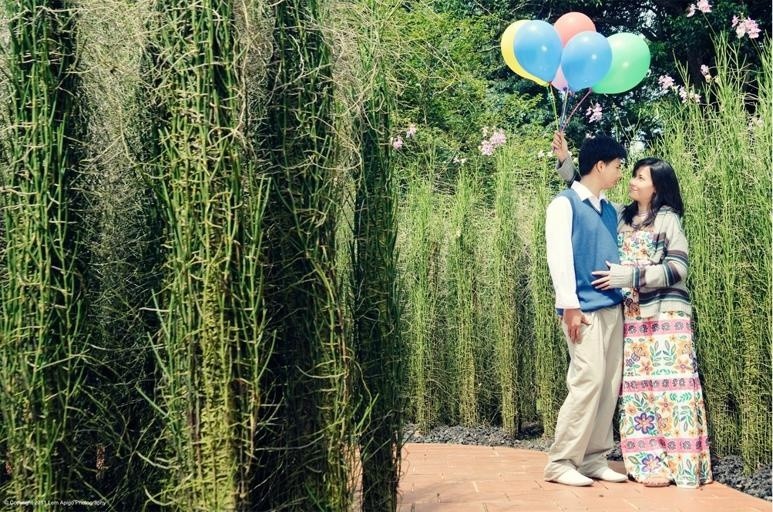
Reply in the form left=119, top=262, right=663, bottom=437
left=587, top=468, right=628, bottom=482
left=551, top=468, right=593, bottom=486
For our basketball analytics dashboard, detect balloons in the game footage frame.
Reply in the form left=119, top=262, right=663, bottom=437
left=499, top=20, right=548, bottom=87
left=561, top=32, right=612, bottom=95
left=553, top=12, right=596, bottom=49
left=513, top=21, right=562, bottom=84
left=589, top=33, right=650, bottom=95
left=550, top=66, right=567, bottom=92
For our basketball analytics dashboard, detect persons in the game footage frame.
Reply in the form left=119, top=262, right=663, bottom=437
left=545, top=136, right=628, bottom=486
left=552, top=131, right=713, bottom=490
